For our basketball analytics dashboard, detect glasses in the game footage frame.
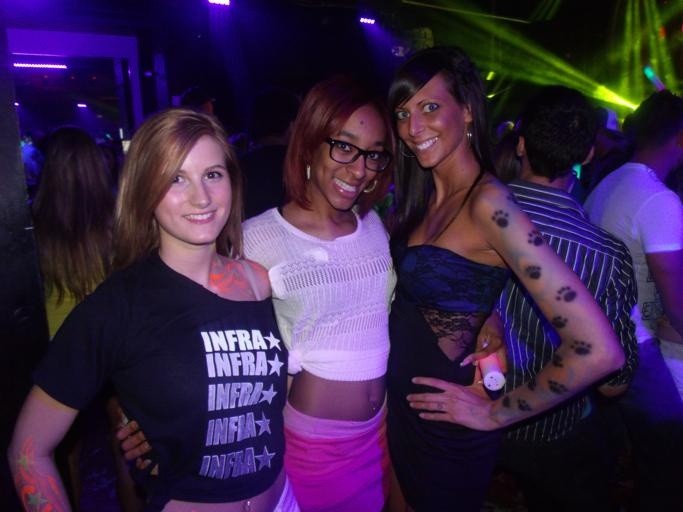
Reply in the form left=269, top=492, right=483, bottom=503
left=324, top=136, right=392, bottom=171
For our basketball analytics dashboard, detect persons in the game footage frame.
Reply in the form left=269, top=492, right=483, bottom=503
left=116, top=77, right=509, bottom=512
left=388, top=46, right=625, bottom=512
left=24, top=127, right=139, bottom=512
left=492, top=83, right=682, bottom=511
left=7, top=110, right=301, bottom=512
left=191, top=91, right=393, bottom=218
